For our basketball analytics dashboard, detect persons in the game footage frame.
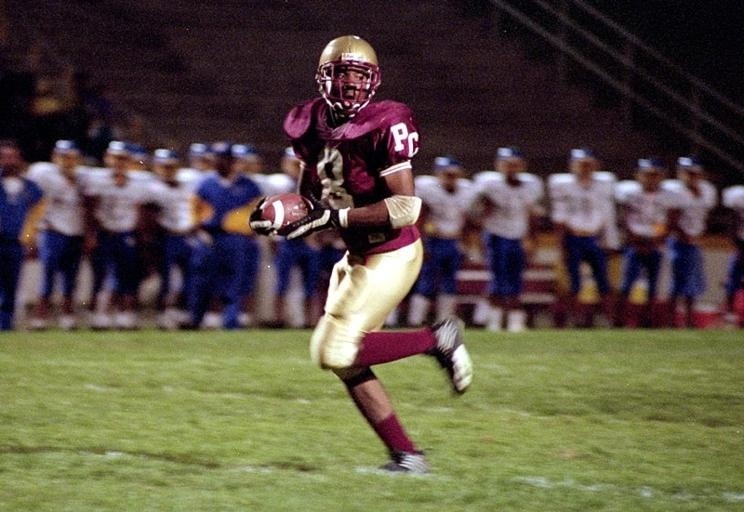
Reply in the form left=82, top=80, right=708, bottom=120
left=251, top=33, right=472, bottom=478
left=0, top=50, right=743, bottom=334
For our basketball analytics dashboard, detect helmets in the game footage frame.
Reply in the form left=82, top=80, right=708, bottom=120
left=493, top=145, right=527, bottom=166
left=151, top=147, right=180, bottom=164
left=674, top=156, right=701, bottom=173
left=51, top=138, right=82, bottom=156
left=186, top=142, right=211, bottom=159
left=105, top=139, right=127, bottom=157
left=432, top=155, right=464, bottom=171
left=231, top=141, right=258, bottom=159
left=211, top=140, right=234, bottom=156
left=567, top=146, right=598, bottom=164
left=636, top=157, right=664, bottom=176
left=315, top=34, right=381, bottom=117
left=126, top=143, right=149, bottom=161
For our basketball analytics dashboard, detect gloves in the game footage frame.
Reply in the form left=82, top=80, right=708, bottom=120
left=247, top=192, right=278, bottom=240
left=276, top=192, right=351, bottom=241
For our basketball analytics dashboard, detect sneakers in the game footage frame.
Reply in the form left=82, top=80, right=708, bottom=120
left=376, top=447, right=431, bottom=477
left=426, top=310, right=474, bottom=395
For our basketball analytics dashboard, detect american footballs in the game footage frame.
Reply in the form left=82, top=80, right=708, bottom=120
left=249, top=193, right=329, bottom=239
